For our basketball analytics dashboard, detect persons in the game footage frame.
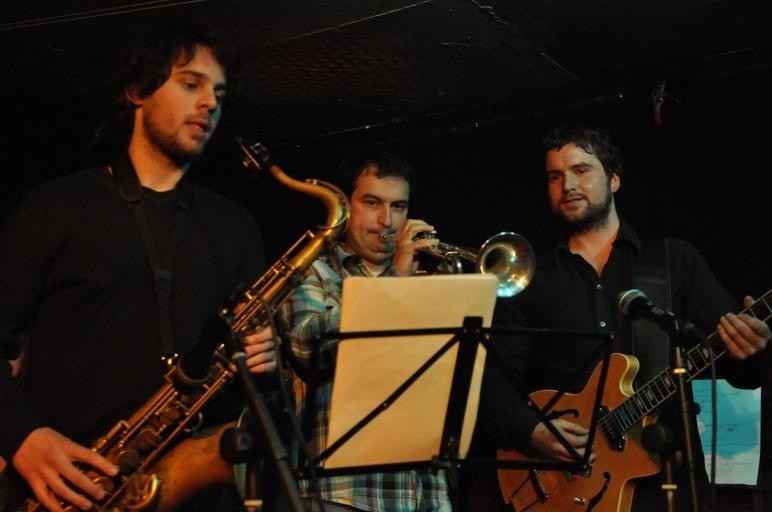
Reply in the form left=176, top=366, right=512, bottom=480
left=1, top=18, right=288, bottom=512
left=483, top=118, right=771, bottom=511
left=275, top=134, right=453, bottom=512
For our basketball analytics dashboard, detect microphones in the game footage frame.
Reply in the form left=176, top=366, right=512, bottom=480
left=616, top=288, right=710, bottom=348
left=173, top=290, right=242, bottom=396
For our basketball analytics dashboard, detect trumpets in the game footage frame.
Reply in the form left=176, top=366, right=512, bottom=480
left=378, top=230, right=535, bottom=298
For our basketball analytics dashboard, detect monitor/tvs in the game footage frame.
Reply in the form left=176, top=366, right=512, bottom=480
left=691, top=373, right=766, bottom=490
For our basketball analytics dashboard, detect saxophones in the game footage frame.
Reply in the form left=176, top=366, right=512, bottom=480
left=16, top=135, right=351, bottom=511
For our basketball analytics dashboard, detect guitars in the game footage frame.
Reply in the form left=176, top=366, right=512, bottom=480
left=496, top=290, right=772, bottom=511
